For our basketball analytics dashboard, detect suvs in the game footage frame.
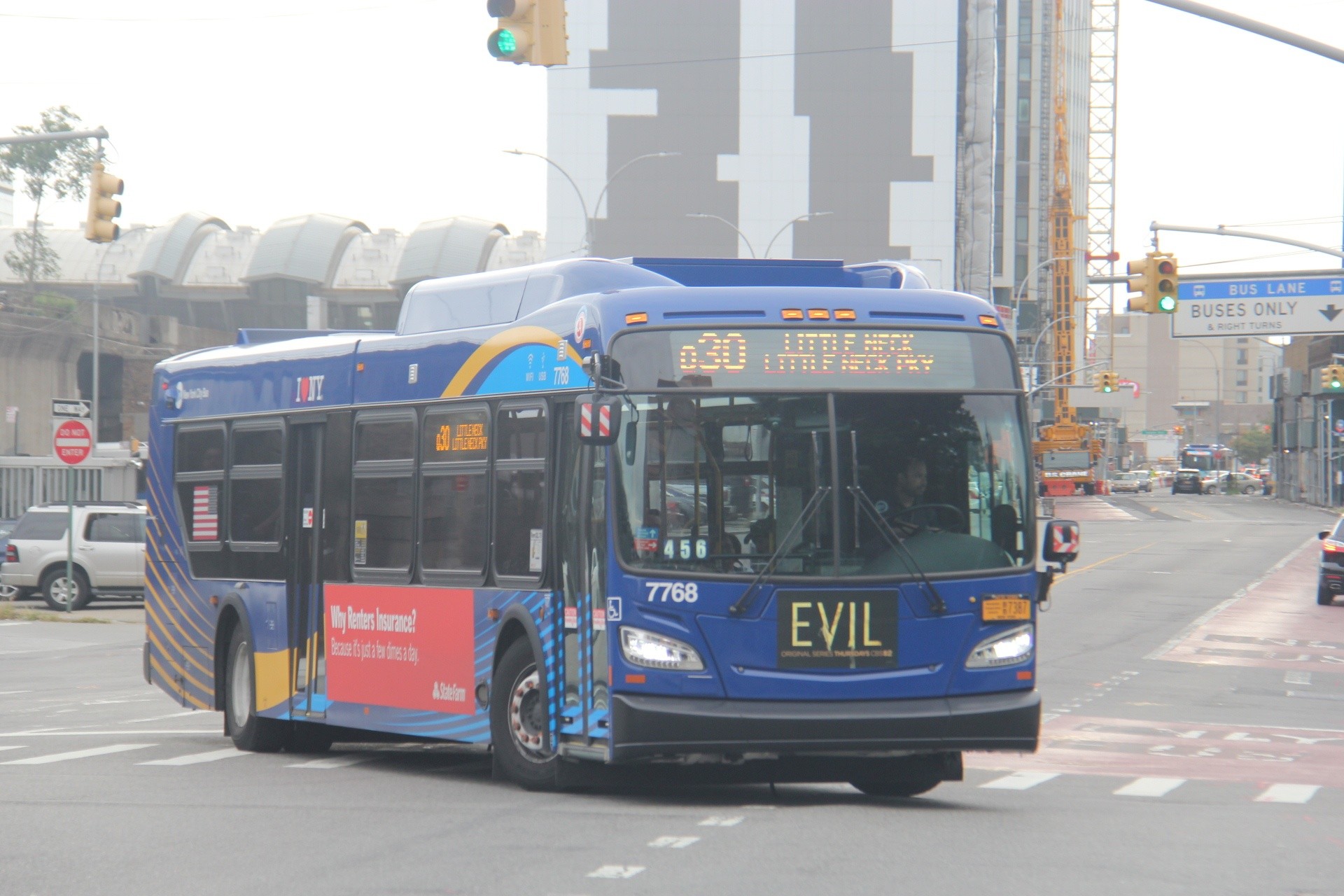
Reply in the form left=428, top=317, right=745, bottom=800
left=2, top=498, right=146, bottom=612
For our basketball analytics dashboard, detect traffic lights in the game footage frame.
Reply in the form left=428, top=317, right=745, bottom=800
left=1150, top=257, right=1178, bottom=314
left=1321, top=367, right=1328, bottom=389
left=1100, top=369, right=1112, bottom=394
left=1328, top=367, right=1344, bottom=389
left=1091, top=373, right=1101, bottom=393
left=1111, top=372, right=1120, bottom=392
left=1174, top=426, right=1184, bottom=435
left=486, top=0, right=571, bottom=69
left=1126, top=258, right=1149, bottom=314
left=82, top=162, right=127, bottom=246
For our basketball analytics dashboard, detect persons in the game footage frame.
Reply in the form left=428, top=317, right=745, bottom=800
left=641, top=509, right=660, bottom=529
left=875, top=457, right=945, bottom=535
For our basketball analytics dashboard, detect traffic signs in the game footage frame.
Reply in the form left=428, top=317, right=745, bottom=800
left=1170, top=276, right=1344, bottom=338
left=50, top=397, right=93, bottom=419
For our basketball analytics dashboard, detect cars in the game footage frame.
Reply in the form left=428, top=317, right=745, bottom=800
left=1110, top=441, right=1272, bottom=495
left=663, top=472, right=1003, bottom=527
left=1317, top=513, right=1344, bottom=604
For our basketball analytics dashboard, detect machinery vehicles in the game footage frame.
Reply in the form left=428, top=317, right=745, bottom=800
left=1036, top=0, right=1096, bottom=496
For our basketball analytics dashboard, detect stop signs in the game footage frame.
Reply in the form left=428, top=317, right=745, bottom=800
left=137, top=254, right=1082, bottom=800
left=51, top=416, right=94, bottom=466
